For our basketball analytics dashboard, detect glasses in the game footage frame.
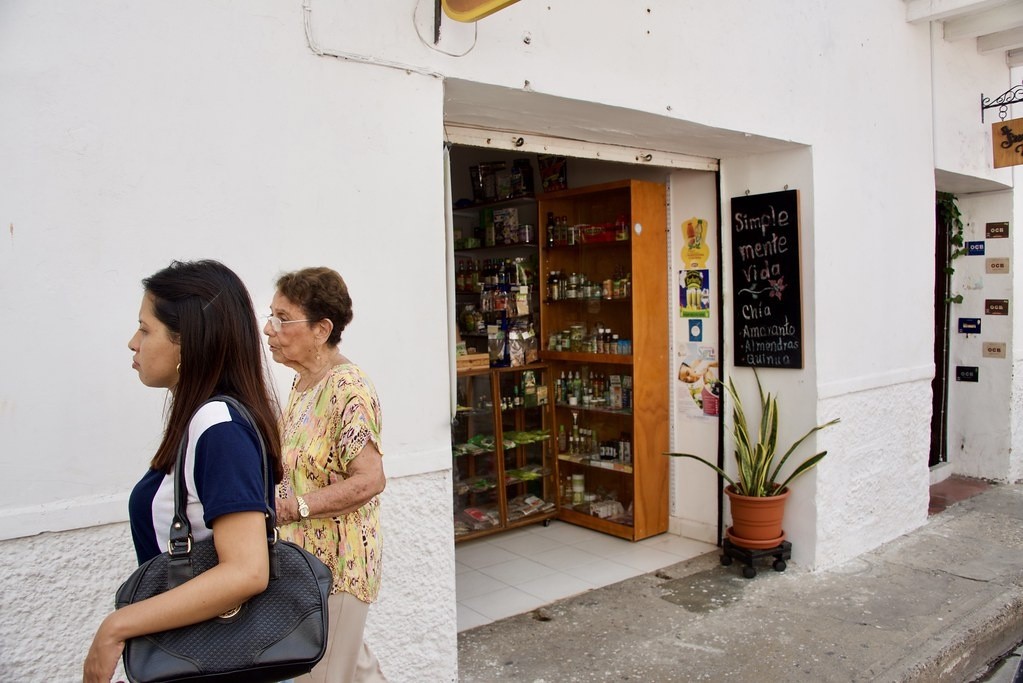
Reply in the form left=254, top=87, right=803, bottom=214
left=258, top=314, right=311, bottom=332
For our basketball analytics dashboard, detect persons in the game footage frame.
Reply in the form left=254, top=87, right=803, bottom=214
left=264, top=267, right=386, bottom=683
left=83, top=259, right=284, bottom=683
left=678, top=357, right=722, bottom=386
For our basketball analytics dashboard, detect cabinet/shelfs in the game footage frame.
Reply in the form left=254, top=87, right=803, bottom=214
left=540, top=179, right=671, bottom=542
left=452, top=363, right=558, bottom=546
left=456, top=196, right=540, bottom=354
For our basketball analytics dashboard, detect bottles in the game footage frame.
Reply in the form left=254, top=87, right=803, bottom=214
left=593, top=326, right=620, bottom=354
left=541, top=212, right=571, bottom=246
left=552, top=369, right=611, bottom=409
left=564, top=476, right=573, bottom=505
left=482, top=384, right=525, bottom=409
left=546, top=271, right=568, bottom=299
left=455, top=255, right=535, bottom=335
left=557, top=423, right=632, bottom=463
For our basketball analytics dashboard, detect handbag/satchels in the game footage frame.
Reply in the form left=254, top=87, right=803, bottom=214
left=115, top=396, right=333, bottom=683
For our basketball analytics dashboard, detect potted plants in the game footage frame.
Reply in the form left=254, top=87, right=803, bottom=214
left=662, top=362, right=857, bottom=548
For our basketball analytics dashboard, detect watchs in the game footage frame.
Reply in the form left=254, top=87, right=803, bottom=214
left=296, top=496, right=309, bottom=517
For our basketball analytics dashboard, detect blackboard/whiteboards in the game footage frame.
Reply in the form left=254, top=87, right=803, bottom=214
left=729, top=188, right=805, bottom=371
